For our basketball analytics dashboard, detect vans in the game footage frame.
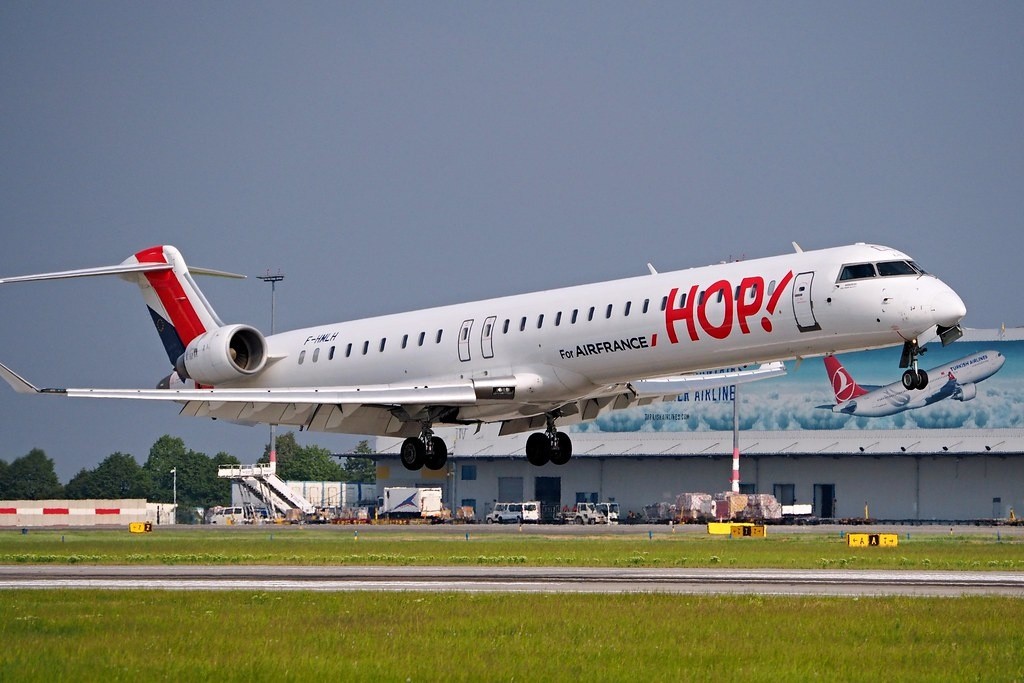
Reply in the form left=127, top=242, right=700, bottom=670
left=210, top=507, right=245, bottom=526
left=498, top=501, right=540, bottom=524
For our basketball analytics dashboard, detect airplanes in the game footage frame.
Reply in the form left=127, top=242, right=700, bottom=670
left=1, top=239, right=969, bottom=471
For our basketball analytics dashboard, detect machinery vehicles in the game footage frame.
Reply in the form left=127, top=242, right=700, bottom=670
left=377, top=485, right=443, bottom=520
left=553, top=501, right=622, bottom=526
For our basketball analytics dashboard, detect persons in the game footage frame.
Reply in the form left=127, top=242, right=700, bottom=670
left=561, top=503, right=642, bottom=526
